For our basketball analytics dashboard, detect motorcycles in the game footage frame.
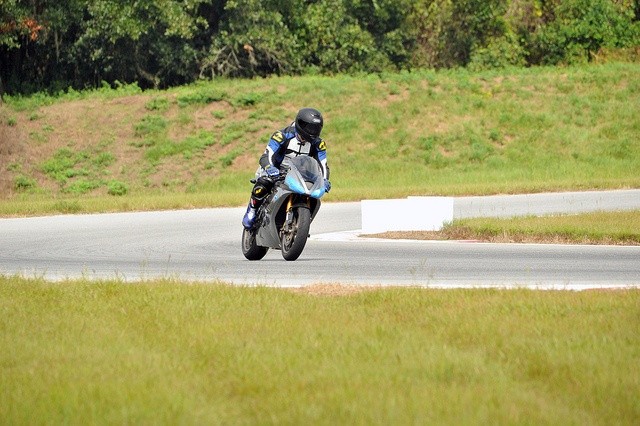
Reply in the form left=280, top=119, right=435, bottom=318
left=242, top=155, right=330, bottom=260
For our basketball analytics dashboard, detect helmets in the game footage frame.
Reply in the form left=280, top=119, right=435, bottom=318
left=295, top=108, right=323, bottom=145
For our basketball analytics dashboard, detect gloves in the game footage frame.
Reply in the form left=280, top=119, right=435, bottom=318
left=265, top=165, right=280, bottom=178
left=324, top=179, right=331, bottom=192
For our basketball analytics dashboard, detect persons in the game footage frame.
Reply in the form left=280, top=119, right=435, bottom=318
left=241, top=108, right=330, bottom=231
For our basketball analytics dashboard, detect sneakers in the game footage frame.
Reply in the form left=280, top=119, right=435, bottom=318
left=242, top=203, right=258, bottom=229
